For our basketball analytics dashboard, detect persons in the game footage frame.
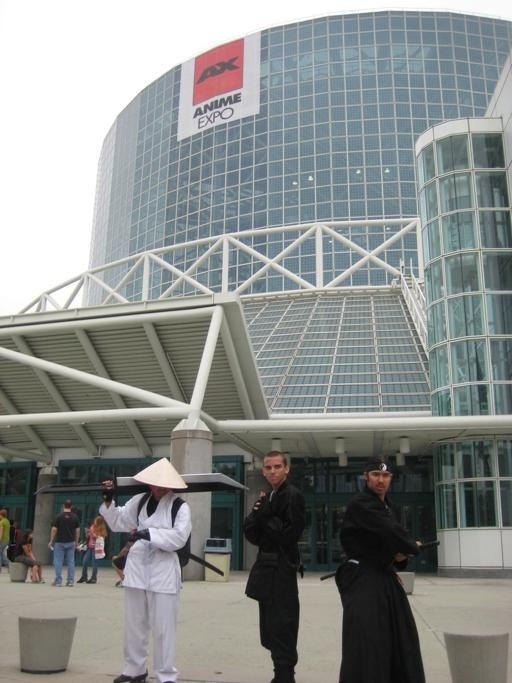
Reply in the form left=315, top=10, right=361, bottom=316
left=334, top=456, right=426, bottom=683
left=0, top=509, right=45, bottom=585
left=98, top=457, right=191, bottom=683
left=48, top=499, right=81, bottom=587
left=110, top=529, right=138, bottom=587
left=240, top=451, right=307, bottom=683
left=76, top=515, right=107, bottom=584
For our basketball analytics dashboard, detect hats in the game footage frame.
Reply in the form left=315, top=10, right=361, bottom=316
left=132, top=458, right=188, bottom=490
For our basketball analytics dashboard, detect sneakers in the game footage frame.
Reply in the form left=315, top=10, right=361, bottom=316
left=52, top=582, right=62, bottom=587
left=113, top=669, right=148, bottom=683
left=77, top=577, right=88, bottom=583
left=87, top=577, right=97, bottom=583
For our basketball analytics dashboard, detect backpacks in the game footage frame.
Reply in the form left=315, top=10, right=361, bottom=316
left=136, top=492, right=190, bottom=566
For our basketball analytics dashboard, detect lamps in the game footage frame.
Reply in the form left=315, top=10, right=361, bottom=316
left=271, top=437, right=410, bottom=468
